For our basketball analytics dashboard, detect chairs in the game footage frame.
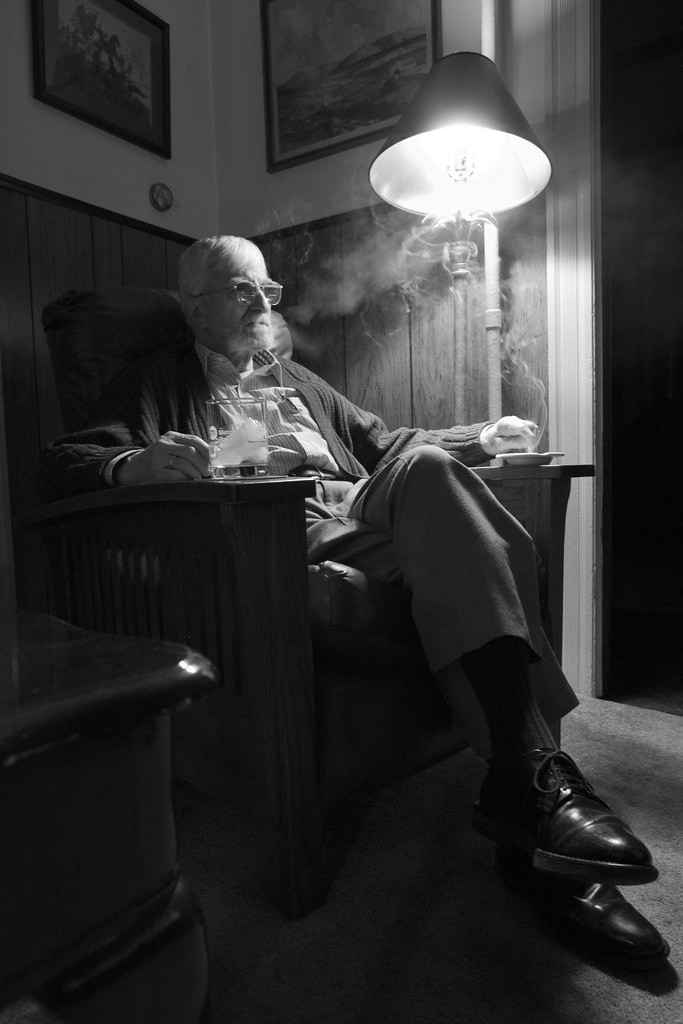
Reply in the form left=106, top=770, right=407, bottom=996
left=10, top=286, right=596, bottom=919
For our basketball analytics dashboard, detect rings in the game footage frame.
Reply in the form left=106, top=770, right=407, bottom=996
left=170, top=455, right=177, bottom=469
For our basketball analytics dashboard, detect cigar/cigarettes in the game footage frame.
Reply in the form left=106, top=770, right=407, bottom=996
left=527, top=443, right=532, bottom=453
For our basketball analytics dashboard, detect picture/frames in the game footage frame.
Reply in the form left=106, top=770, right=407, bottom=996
left=260, top=0, right=443, bottom=174
left=32, top=0, right=171, bottom=160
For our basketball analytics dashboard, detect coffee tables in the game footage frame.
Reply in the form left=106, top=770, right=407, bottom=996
left=0, top=608, right=231, bottom=1024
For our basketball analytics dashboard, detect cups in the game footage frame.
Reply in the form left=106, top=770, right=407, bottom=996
left=205, top=398, right=269, bottom=480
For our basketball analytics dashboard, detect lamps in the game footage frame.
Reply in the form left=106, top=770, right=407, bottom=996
left=369, top=50, right=554, bottom=425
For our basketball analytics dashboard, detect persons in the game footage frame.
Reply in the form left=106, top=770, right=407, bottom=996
left=39, top=236, right=670, bottom=964
